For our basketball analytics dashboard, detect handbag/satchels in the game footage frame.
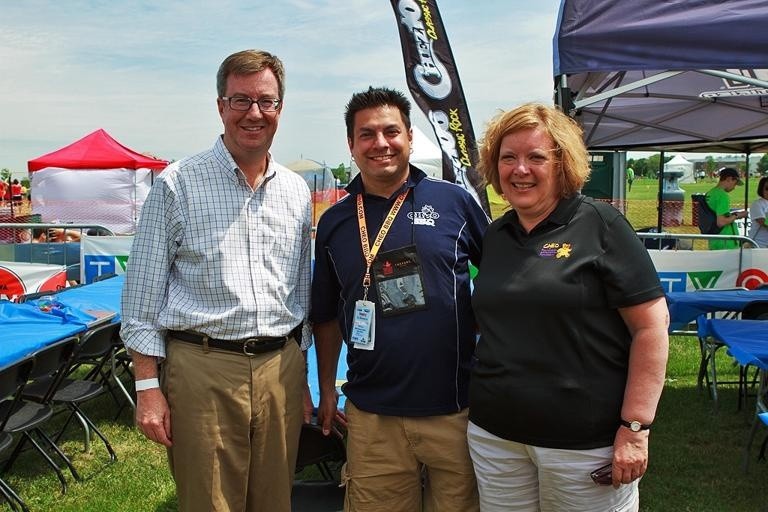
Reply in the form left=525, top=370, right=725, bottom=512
left=691, top=195, right=729, bottom=234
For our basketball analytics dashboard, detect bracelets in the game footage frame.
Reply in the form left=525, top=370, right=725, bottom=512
left=132, top=378, right=162, bottom=394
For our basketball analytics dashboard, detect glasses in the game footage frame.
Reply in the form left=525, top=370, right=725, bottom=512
left=589, top=463, right=612, bottom=487
left=222, top=95, right=281, bottom=112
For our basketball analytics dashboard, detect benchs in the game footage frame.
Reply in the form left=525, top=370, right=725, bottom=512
left=9, top=239, right=81, bottom=281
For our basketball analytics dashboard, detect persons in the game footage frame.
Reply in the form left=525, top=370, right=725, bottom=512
left=465, top=103, right=671, bottom=512
left=119, top=49, right=310, bottom=511
left=21, top=224, right=85, bottom=243
left=747, top=175, right=768, bottom=249
left=310, top=84, right=493, bottom=512
left=704, top=167, right=746, bottom=251
left=11, top=181, right=26, bottom=215
left=624, top=161, right=767, bottom=190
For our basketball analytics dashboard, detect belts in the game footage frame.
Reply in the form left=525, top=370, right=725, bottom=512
left=166, top=329, right=292, bottom=357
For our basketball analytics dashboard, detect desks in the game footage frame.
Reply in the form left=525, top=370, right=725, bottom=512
left=666, top=284, right=766, bottom=473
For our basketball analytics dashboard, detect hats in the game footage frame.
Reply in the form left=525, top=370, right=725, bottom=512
left=720, top=167, right=743, bottom=186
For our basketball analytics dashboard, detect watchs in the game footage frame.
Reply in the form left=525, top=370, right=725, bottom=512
left=622, top=419, right=651, bottom=432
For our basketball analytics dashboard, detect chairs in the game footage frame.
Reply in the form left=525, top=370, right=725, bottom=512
left=0, top=271, right=143, bottom=511
